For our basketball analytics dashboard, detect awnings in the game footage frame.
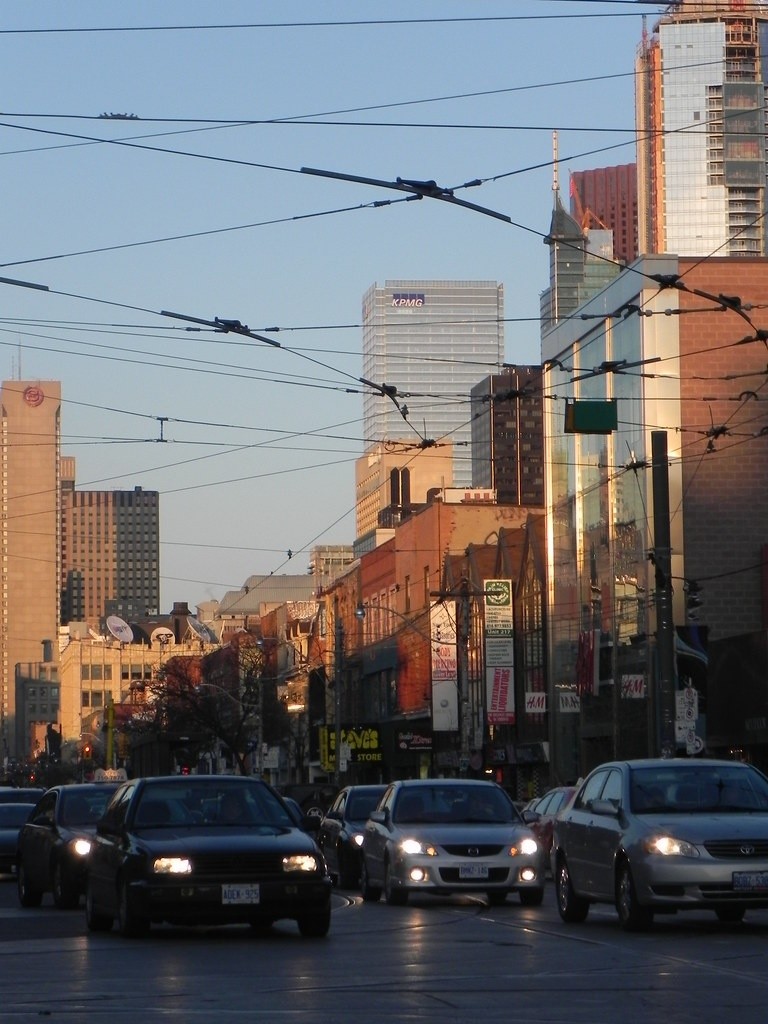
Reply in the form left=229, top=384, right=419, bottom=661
left=390, top=719, right=442, bottom=753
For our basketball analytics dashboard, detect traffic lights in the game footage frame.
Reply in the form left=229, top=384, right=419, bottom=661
left=83, top=743, right=92, bottom=760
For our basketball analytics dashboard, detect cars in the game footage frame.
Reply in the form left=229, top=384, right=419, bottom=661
left=525, top=786, right=577, bottom=869
left=318, top=784, right=451, bottom=891
left=87, top=775, right=331, bottom=941
left=364, top=779, right=543, bottom=907
left=17, top=768, right=130, bottom=910
left=549, top=756, right=768, bottom=933
left=0, top=802, right=37, bottom=868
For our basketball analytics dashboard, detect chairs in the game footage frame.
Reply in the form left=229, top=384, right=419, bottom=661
left=354, top=799, right=371, bottom=822
left=450, top=799, right=475, bottom=823
left=635, top=786, right=668, bottom=812
left=399, top=795, right=429, bottom=824
left=63, top=797, right=94, bottom=823
left=138, top=799, right=175, bottom=828
left=675, top=786, right=708, bottom=803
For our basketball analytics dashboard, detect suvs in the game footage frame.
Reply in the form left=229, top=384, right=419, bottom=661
left=278, top=784, right=339, bottom=840
left=0, top=787, right=50, bottom=805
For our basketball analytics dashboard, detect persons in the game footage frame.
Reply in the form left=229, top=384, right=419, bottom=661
left=221, top=793, right=243, bottom=821
left=464, top=793, right=483, bottom=818
left=641, top=787, right=666, bottom=810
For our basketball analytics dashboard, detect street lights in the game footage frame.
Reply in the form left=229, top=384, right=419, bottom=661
left=353, top=601, right=470, bottom=780
left=193, top=683, right=263, bottom=777
left=253, top=637, right=341, bottom=789
